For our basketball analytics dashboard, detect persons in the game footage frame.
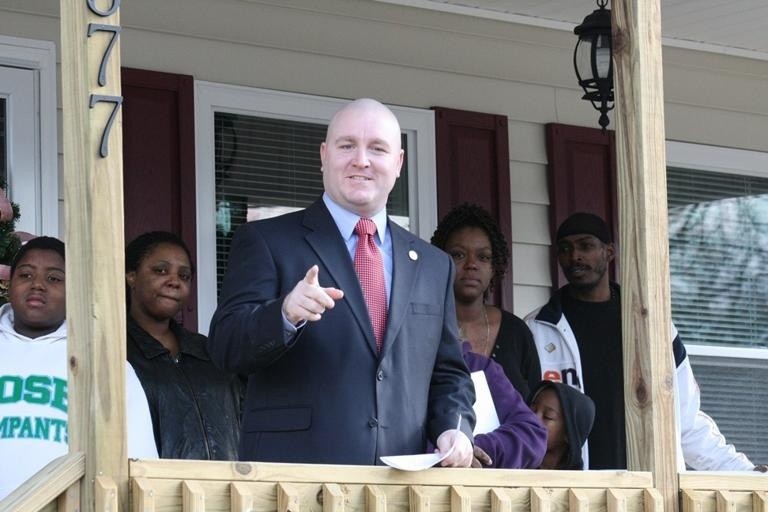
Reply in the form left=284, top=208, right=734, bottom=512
left=115, top=228, right=252, bottom=462
left=519, top=377, right=599, bottom=469
left=450, top=334, right=549, bottom=470
left=204, top=96, right=481, bottom=469
left=0, top=234, right=163, bottom=503
left=520, top=210, right=768, bottom=471
left=416, top=201, right=544, bottom=412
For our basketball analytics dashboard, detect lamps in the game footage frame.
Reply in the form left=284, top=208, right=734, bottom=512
left=573, top=1, right=614, bottom=134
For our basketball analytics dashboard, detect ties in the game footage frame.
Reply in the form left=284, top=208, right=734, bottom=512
left=354, top=218, right=388, bottom=355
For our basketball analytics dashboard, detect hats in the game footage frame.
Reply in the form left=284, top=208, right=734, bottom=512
left=557, top=212, right=612, bottom=245
